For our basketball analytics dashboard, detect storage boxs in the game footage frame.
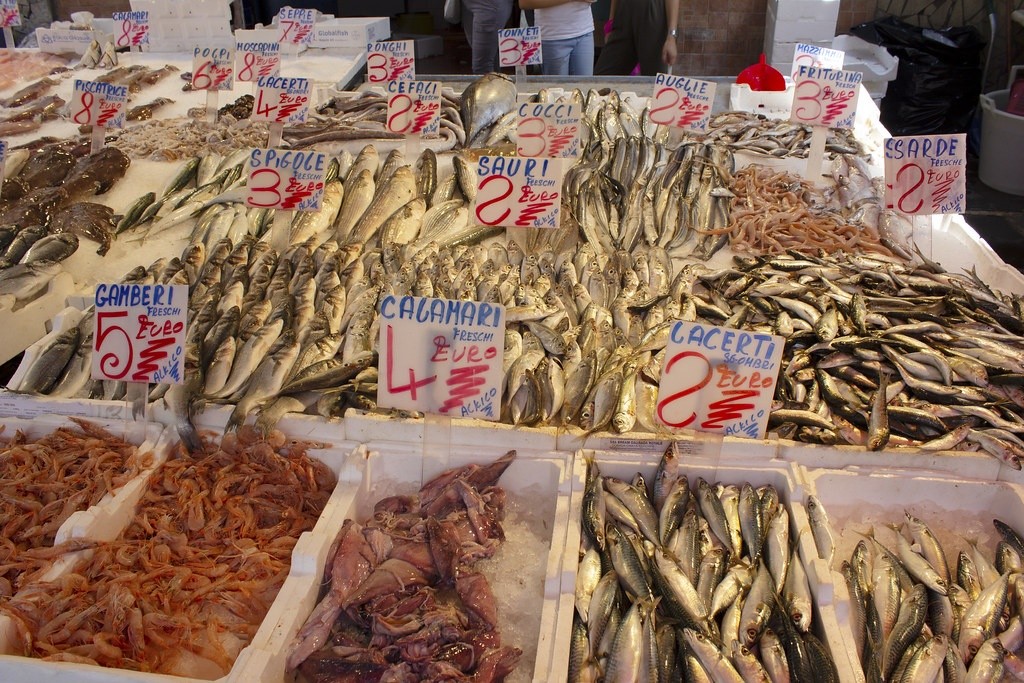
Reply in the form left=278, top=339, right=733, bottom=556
left=0, top=417, right=1024, bottom=682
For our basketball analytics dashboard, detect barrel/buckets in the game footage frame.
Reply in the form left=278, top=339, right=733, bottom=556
left=978, top=88, right=1024, bottom=196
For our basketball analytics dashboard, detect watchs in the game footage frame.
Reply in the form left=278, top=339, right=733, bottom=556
left=667, top=28, right=679, bottom=39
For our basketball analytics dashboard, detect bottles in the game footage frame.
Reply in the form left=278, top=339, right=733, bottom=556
left=1006, top=78, right=1024, bottom=117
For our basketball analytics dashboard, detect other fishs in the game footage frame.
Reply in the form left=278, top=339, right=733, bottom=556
left=0, top=41, right=1024, bottom=683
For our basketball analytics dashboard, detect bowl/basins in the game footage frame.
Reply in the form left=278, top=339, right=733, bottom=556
left=736, top=53, right=787, bottom=91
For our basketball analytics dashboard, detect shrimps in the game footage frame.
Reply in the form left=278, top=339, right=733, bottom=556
left=0, top=416, right=334, bottom=676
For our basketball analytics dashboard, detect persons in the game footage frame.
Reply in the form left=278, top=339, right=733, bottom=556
left=459, top=0, right=514, bottom=74
left=519, top=0, right=681, bottom=75
